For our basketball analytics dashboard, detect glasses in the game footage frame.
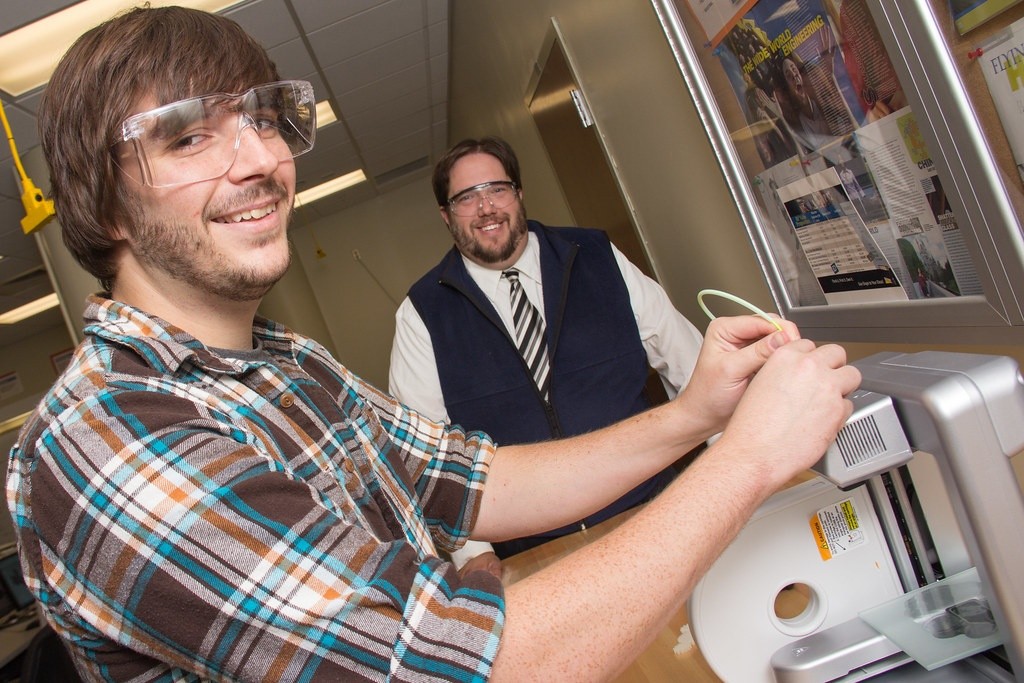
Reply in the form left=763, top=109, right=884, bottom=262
left=110, top=80, right=316, bottom=188
left=446, top=180, right=519, bottom=216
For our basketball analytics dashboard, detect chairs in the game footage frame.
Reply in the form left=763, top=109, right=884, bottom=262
left=11, top=623, right=84, bottom=683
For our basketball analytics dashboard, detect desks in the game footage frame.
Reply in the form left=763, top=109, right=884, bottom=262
left=0, top=601, right=42, bottom=670
left=499, top=468, right=824, bottom=683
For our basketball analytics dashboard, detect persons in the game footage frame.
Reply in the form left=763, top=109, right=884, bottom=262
left=726, top=0, right=907, bottom=188
left=918, top=267, right=930, bottom=298
left=836, top=152, right=867, bottom=220
left=5, top=9, right=862, bottom=683
left=388, top=136, right=728, bottom=580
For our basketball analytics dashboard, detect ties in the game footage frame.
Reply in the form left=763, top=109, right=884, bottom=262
left=501, top=270, right=549, bottom=402
